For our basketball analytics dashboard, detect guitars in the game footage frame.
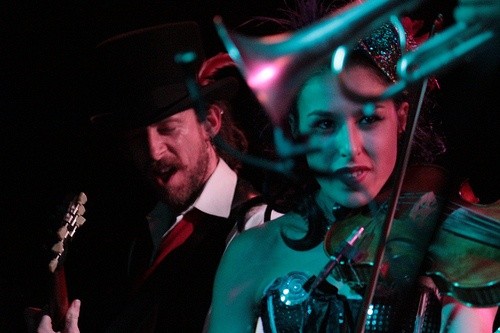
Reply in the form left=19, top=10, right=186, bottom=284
left=23, top=192, right=87, bottom=333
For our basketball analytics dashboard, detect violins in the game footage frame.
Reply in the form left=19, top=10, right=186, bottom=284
left=323, top=191, right=500, bottom=309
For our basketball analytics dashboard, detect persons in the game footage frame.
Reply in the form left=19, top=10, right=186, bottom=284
left=208, top=0, right=500, bottom=333
left=24, top=21, right=286, bottom=333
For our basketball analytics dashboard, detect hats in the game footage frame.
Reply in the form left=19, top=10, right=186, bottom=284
left=89, top=21, right=237, bottom=130
left=358, top=16, right=407, bottom=94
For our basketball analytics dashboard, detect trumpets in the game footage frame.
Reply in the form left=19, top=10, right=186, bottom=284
left=199, top=0, right=500, bottom=125
